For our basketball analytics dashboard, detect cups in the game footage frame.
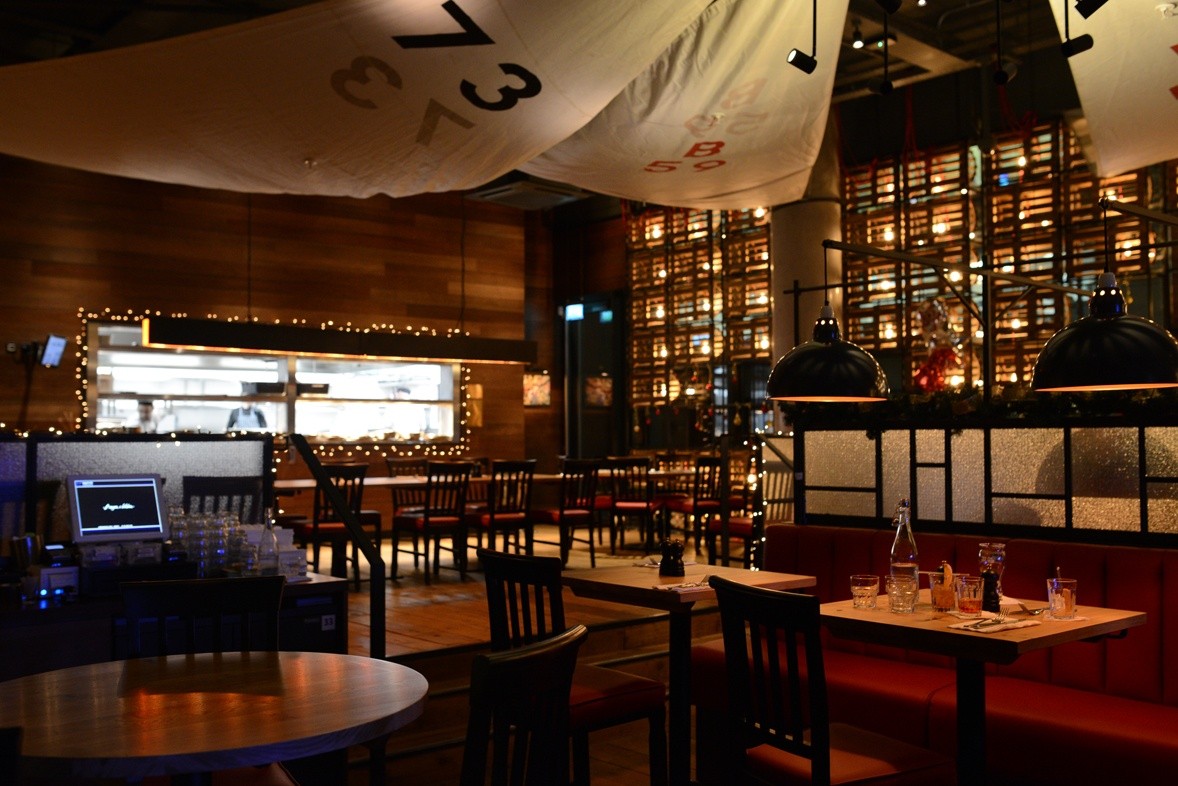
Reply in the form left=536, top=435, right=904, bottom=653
left=851, top=575, right=880, bottom=610
left=1047, top=579, right=1077, bottom=620
left=281, top=557, right=301, bottom=579
left=956, top=577, right=984, bottom=618
left=886, top=575, right=917, bottom=614
left=928, top=573, right=955, bottom=613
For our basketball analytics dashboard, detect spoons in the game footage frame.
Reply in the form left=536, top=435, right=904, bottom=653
left=1019, top=604, right=1050, bottom=616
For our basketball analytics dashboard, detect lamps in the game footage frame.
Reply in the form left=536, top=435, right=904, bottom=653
left=1029, top=208, right=1178, bottom=392
left=764, top=248, right=890, bottom=402
left=992, top=0, right=1109, bottom=85
left=851, top=19, right=864, bottom=50
left=786, top=0, right=818, bottom=74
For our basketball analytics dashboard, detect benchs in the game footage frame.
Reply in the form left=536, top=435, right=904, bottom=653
left=690, top=523, right=1178, bottom=786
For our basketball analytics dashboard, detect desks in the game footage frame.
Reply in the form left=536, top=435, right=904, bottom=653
left=0, top=651, right=429, bottom=786
left=561, top=558, right=818, bottom=786
left=272, top=466, right=716, bottom=580
left=818, top=587, right=1147, bottom=786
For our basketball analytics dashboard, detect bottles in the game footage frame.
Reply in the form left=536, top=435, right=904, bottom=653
left=258, top=508, right=279, bottom=576
left=890, top=498, right=920, bottom=606
left=975, top=541, right=1007, bottom=611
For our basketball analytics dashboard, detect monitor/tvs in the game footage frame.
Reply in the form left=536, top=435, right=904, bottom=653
left=65, top=473, right=170, bottom=541
left=39, top=335, right=68, bottom=367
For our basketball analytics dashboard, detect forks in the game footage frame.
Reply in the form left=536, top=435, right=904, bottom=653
left=976, top=608, right=1010, bottom=628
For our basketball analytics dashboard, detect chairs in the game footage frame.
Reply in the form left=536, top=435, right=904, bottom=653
left=116, top=546, right=933, bottom=786
left=606, top=453, right=757, bottom=547
left=281, top=463, right=371, bottom=588
left=390, top=459, right=474, bottom=585
left=181, top=472, right=264, bottom=522
left=450, top=456, right=494, bottom=564
left=387, top=457, right=453, bottom=567
left=530, top=457, right=601, bottom=570
left=270, top=461, right=308, bottom=553
left=461, top=457, right=539, bottom=557
left=320, top=459, right=382, bottom=555
left=0, top=479, right=62, bottom=584
